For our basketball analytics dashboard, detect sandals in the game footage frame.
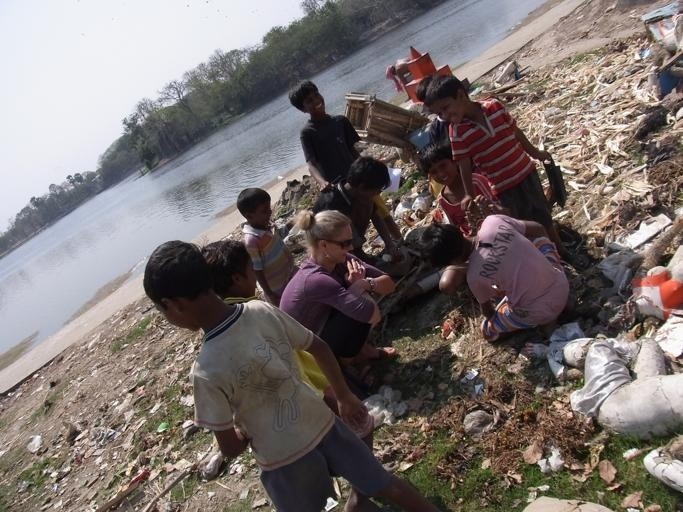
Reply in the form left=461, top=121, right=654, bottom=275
left=360, top=346, right=395, bottom=364
left=339, top=366, right=377, bottom=396
left=556, top=245, right=590, bottom=273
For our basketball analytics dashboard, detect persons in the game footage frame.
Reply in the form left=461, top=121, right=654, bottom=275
left=237, top=187, right=300, bottom=307
left=386, top=57, right=411, bottom=92
left=424, top=74, right=562, bottom=255
left=143, top=240, right=440, bottom=512
left=312, top=155, right=399, bottom=265
left=414, top=75, right=452, bottom=145
left=200, top=240, right=384, bottom=512
left=278, top=210, right=396, bottom=371
left=418, top=214, right=569, bottom=342
left=417, top=140, right=514, bottom=296
left=289, top=80, right=405, bottom=248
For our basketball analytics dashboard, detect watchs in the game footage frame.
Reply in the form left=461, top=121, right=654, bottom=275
left=366, top=277, right=376, bottom=299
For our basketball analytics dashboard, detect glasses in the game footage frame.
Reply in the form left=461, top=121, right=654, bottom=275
left=317, top=238, right=355, bottom=249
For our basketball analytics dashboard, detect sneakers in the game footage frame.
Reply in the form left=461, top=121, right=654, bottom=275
left=569, top=273, right=589, bottom=301
